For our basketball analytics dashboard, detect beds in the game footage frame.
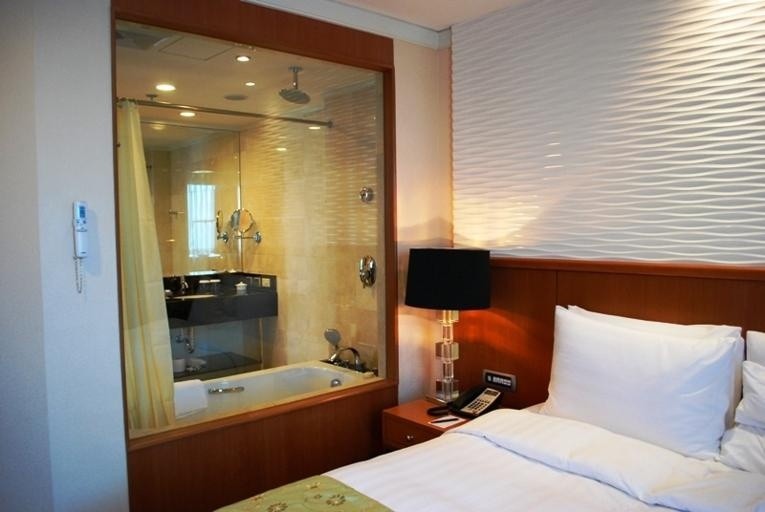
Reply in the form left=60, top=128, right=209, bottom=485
left=217, top=261, right=765, bottom=512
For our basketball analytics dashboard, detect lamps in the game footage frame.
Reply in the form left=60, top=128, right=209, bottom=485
left=405, top=247, right=489, bottom=405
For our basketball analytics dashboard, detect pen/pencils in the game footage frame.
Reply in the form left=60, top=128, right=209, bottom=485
left=432, top=418, right=458, bottom=423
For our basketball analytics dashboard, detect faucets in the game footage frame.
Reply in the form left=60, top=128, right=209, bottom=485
left=329, top=347, right=364, bottom=371
left=180, top=274, right=189, bottom=289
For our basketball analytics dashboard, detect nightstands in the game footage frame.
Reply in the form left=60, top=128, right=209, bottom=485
left=382, top=397, right=499, bottom=450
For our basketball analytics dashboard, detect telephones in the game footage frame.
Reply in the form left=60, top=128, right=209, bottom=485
left=448, top=383, right=503, bottom=419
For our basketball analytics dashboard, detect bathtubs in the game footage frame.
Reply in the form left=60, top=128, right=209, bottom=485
left=127, top=360, right=374, bottom=440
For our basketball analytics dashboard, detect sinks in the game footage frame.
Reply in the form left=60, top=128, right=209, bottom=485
left=171, top=290, right=222, bottom=297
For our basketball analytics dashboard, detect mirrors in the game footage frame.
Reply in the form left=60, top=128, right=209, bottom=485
left=230, top=210, right=262, bottom=244
left=141, top=123, right=242, bottom=275
left=213, top=211, right=230, bottom=243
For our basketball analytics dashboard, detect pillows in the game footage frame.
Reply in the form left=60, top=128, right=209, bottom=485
left=538, top=304, right=765, bottom=471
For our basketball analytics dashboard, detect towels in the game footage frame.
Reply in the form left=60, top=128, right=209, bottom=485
left=175, top=379, right=208, bottom=420
